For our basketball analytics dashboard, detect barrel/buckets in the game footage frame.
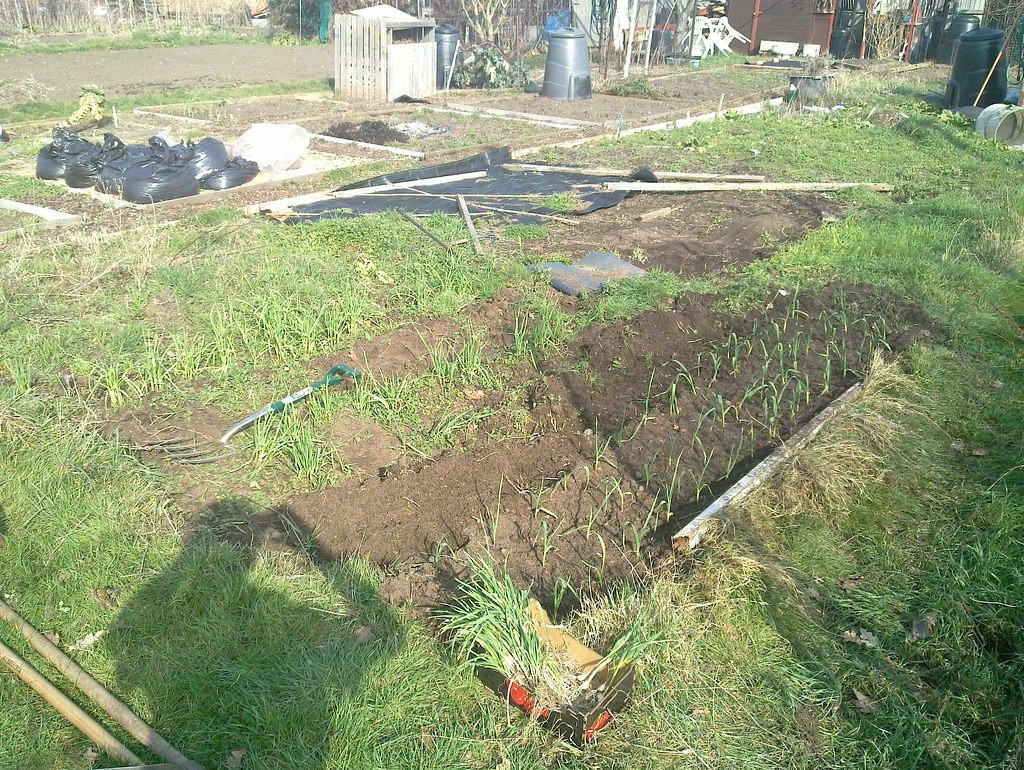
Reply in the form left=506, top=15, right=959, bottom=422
left=976, top=103, right=1024, bottom=149
